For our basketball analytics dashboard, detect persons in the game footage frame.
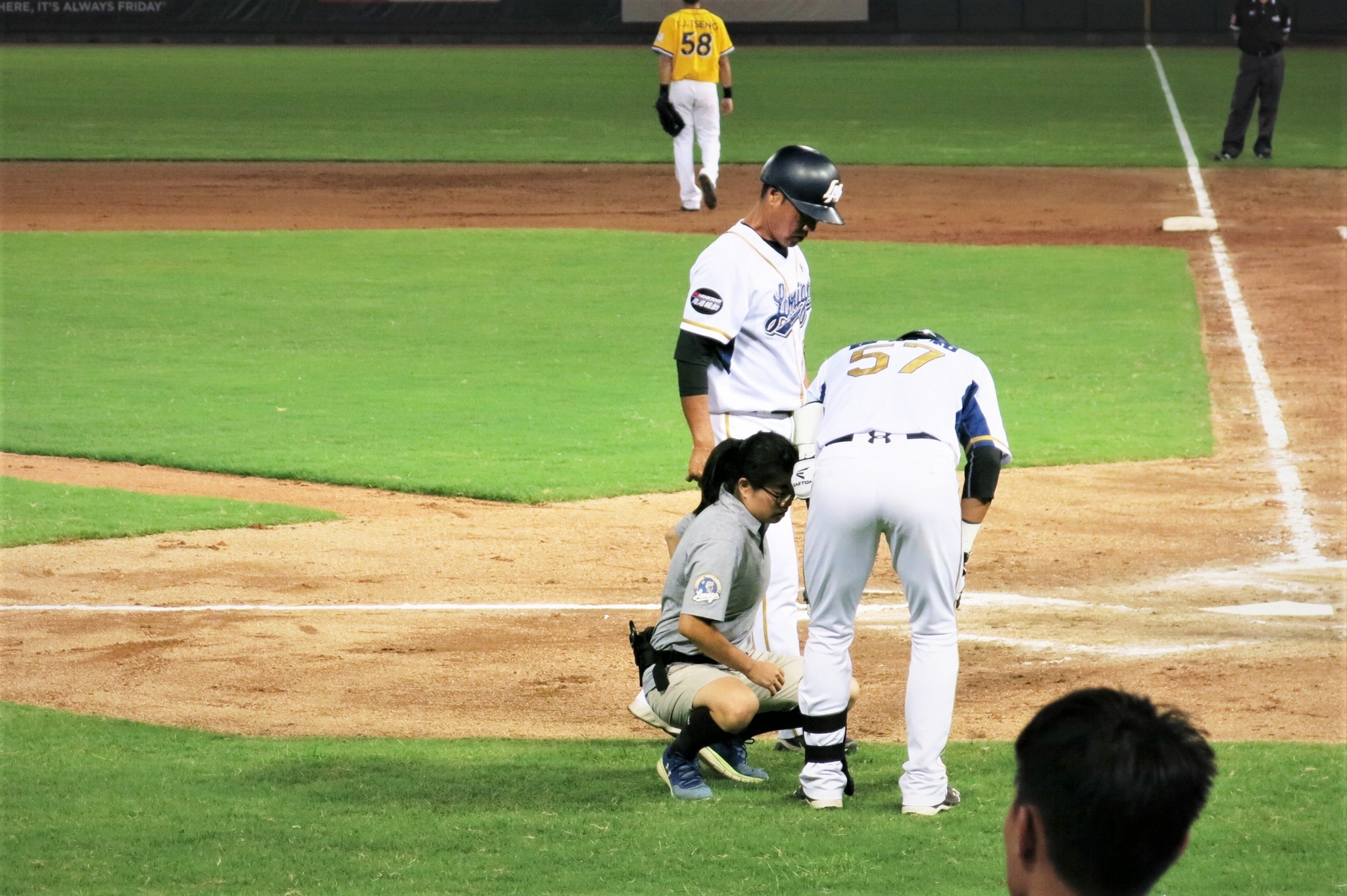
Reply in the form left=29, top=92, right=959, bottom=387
left=791, top=329, right=1012, bottom=815
left=1003, top=687, right=1220, bottom=896
left=628, top=145, right=860, bottom=801
left=651, top=0, right=735, bottom=212
left=1213, top=0, right=1291, bottom=162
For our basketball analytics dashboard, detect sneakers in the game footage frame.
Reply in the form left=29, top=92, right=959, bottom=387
left=792, top=784, right=843, bottom=810
left=772, top=734, right=857, bottom=755
left=698, top=738, right=769, bottom=783
left=902, top=786, right=961, bottom=816
left=627, top=689, right=682, bottom=737
left=655, top=744, right=715, bottom=803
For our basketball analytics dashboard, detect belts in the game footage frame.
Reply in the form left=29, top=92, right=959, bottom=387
left=771, top=411, right=793, bottom=417
left=824, top=433, right=941, bottom=447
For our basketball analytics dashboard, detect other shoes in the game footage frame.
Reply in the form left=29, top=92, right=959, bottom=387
left=699, top=174, right=718, bottom=210
left=1214, top=151, right=1234, bottom=161
left=680, top=205, right=698, bottom=212
left=1257, top=149, right=1273, bottom=159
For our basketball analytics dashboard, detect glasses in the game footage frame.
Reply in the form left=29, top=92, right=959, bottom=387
left=761, top=486, right=798, bottom=503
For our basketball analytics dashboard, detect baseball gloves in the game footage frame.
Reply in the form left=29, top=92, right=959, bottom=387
left=654, top=95, right=688, bottom=136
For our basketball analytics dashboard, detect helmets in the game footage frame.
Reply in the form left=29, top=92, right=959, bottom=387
left=898, top=329, right=948, bottom=344
left=759, top=144, right=843, bottom=225
